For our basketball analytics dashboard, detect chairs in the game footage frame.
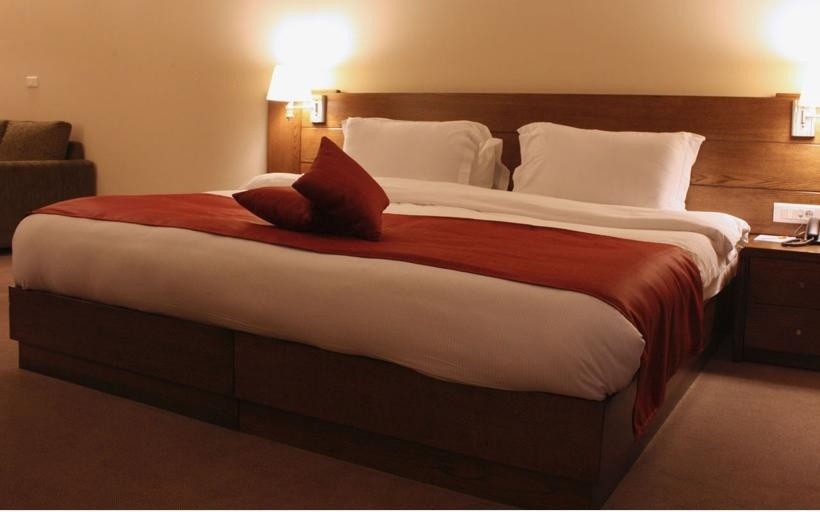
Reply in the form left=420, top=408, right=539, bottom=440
left=0, top=119, right=98, bottom=256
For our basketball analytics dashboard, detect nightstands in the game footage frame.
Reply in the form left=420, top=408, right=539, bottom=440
left=734, top=234, right=820, bottom=364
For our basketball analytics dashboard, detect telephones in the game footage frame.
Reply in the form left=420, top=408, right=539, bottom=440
left=804, top=218, right=820, bottom=245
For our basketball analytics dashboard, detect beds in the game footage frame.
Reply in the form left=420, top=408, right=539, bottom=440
left=7, top=80, right=820, bottom=510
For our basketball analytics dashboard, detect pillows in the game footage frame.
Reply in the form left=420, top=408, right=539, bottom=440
left=338, top=116, right=508, bottom=187
left=294, top=136, right=390, bottom=242
left=233, top=190, right=340, bottom=237
left=511, top=121, right=705, bottom=210
